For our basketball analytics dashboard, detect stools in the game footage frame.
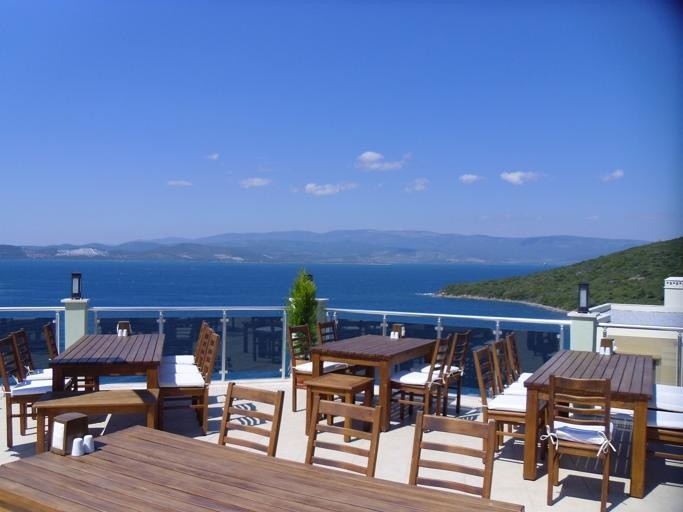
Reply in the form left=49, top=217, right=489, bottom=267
left=302, top=372, right=377, bottom=443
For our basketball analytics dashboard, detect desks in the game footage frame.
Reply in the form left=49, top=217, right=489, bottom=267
left=309, top=333, right=437, bottom=432
left=0, top=424, right=528, bottom=512
left=522, top=348, right=654, bottom=499
left=48, top=334, right=165, bottom=392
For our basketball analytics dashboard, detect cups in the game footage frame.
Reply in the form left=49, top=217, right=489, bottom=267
left=123, top=329, right=128, bottom=336
left=390, top=331, right=394, bottom=339
left=605, top=347, right=611, bottom=355
left=118, top=329, right=122, bottom=336
left=83, top=435, right=95, bottom=453
left=395, top=332, right=399, bottom=339
left=600, top=347, right=605, bottom=354
left=71, top=438, right=84, bottom=457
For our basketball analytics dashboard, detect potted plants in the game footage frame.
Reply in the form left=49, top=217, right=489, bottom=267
left=284, top=266, right=320, bottom=390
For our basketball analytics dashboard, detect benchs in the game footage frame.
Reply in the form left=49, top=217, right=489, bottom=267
left=32, top=389, right=159, bottom=455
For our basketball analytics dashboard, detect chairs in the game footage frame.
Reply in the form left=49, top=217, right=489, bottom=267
left=0, top=334, right=72, bottom=449
left=473, top=344, right=549, bottom=462
left=162, top=320, right=208, bottom=363
left=158, top=334, right=220, bottom=433
left=159, top=327, right=214, bottom=373
left=218, top=382, right=285, bottom=457
left=389, top=331, right=452, bottom=434
left=409, top=329, right=472, bottom=416
left=43, top=322, right=99, bottom=393
left=304, top=395, right=383, bottom=478
left=506, top=332, right=534, bottom=383
left=288, top=323, right=356, bottom=426
left=318, top=320, right=338, bottom=345
left=646, top=410, right=683, bottom=468
left=546, top=373, right=611, bottom=512
left=8, top=327, right=78, bottom=420
left=648, top=397, right=683, bottom=412
left=651, top=383, right=683, bottom=397
left=408, top=410, right=497, bottom=499
left=492, top=338, right=562, bottom=445
